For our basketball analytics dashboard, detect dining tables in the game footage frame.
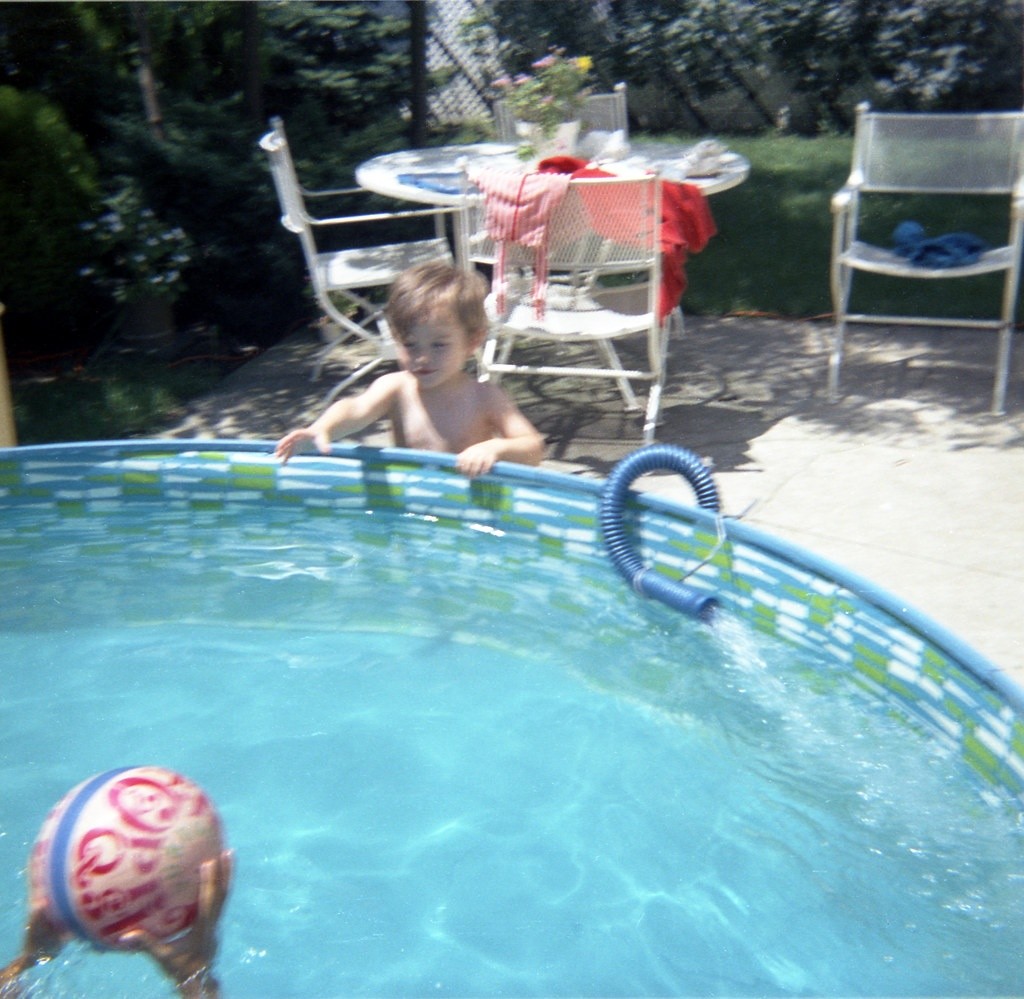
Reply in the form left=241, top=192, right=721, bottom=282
left=357, top=143, right=750, bottom=206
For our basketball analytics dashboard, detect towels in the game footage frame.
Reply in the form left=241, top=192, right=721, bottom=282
left=538, top=155, right=720, bottom=328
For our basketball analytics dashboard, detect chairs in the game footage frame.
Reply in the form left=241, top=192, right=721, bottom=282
left=477, top=172, right=684, bottom=447
left=260, top=117, right=470, bottom=380
left=826, top=101, right=1024, bottom=416
left=495, top=82, right=628, bottom=142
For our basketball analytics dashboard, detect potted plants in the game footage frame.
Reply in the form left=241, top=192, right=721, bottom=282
left=491, top=45, right=597, bottom=160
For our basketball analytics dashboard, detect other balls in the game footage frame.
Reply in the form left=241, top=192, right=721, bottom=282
left=29, top=764, right=223, bottom=953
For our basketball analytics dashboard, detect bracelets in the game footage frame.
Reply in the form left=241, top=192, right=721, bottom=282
left=172, top=960, right=214, bottom=993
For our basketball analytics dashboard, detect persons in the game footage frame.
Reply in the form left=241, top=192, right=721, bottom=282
left=272, top=260, right=546, bottom=478
left=3, top=844, right=236, bottom=999
